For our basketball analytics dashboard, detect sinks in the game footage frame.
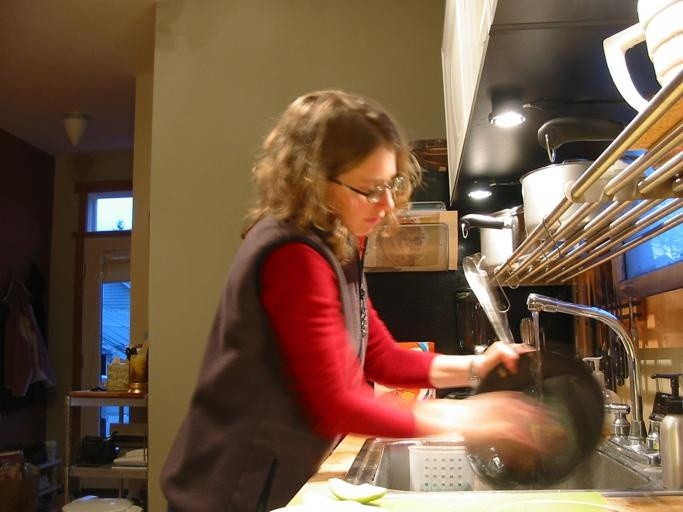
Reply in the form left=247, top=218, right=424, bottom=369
left=344, top=428, right=656, bottom=495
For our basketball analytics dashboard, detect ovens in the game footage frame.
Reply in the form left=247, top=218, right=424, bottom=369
left=361, top=201, right=457, bottom=273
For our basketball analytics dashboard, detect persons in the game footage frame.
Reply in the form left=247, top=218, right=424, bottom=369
left=154, top=86, right=565, bottom=509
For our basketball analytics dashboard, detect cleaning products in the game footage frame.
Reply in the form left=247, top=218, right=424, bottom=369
left=652, top=371, right=683, bottom=490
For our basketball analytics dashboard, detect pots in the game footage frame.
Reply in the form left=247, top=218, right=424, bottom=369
left=458, top=252, right=612, bottom=488
left=460, top=160, right=597, bottom=276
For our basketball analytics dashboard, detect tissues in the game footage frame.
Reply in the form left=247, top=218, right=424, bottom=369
left=107, top=357, right=129, bottom=391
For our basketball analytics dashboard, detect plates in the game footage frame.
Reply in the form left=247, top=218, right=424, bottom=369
left=62, top=497, right=131, bottom=511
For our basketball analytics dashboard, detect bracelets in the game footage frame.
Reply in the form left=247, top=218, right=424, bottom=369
left=464, top=355, right=481, bottom=390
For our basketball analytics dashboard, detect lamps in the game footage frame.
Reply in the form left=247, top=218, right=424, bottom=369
left=63, top=111, right=90, bottom=148
left=488, top=100, right=529, bottom=128
left=467, top=182, right=490, bottom=201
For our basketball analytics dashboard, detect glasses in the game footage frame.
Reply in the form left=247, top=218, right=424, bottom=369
left=326, top=175, right=407, bottom=204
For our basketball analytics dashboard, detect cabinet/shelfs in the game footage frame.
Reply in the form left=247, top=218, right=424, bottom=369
left=64, top=390, right=148, bottom=511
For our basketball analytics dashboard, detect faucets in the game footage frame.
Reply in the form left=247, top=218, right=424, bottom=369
left=524, top=291, right=648, bottom=441
